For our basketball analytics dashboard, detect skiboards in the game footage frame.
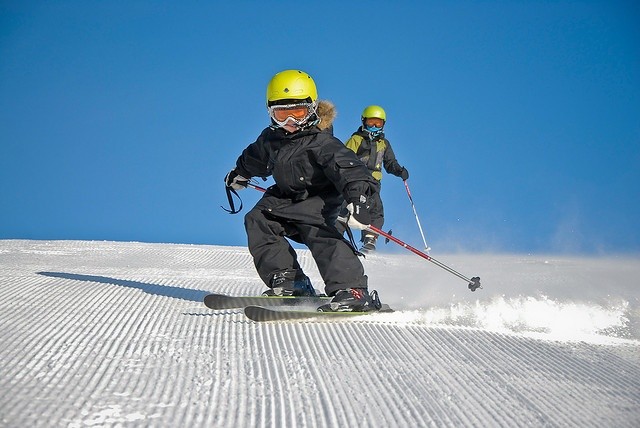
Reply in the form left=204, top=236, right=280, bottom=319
left=360, top=243, right=376, bottom=254
left=204, top=294, right=395, bottom=322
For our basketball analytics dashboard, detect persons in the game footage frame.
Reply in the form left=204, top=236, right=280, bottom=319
left=225, top=69, right=379, bottom=313
left=335, top=104, right=409, bottom=252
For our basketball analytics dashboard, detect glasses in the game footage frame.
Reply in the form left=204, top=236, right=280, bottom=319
left=365, top=118, right=384, bottom=127
left=268, top=102, right=321, bottom=128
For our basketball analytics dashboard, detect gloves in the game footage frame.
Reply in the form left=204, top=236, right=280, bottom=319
left=224, top=168, right=251, bottom=189
left=340, top=190, right=371, bottom=230
left=398, top=165, right=409, bottom=182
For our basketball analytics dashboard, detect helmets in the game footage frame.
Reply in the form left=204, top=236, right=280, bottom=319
left=361, top=105, right=386, bottom=122
left=266, top=69, right=318, bottom=108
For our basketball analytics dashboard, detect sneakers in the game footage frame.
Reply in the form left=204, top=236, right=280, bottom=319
left=261, top=268, right=307, bottom=296
left=361, top=234, right=378, bottom=250
left=317, top=287, right=373, bottom=314
